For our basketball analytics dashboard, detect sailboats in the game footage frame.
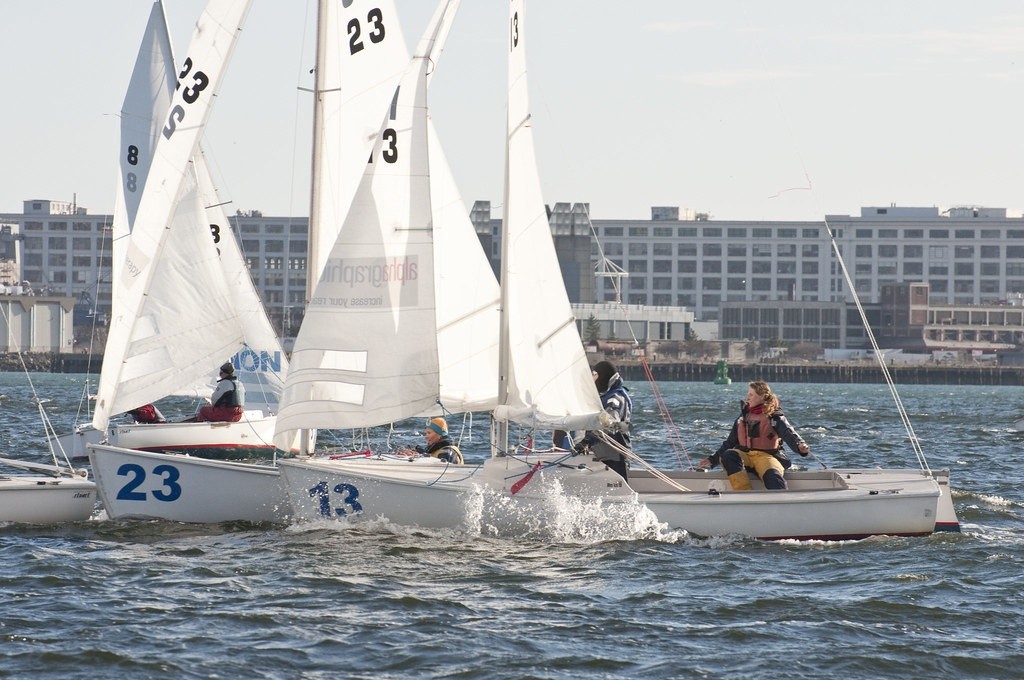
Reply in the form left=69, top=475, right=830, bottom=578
left=29, top=0, right=967, bottom=556
left=0, top=292, right=99, bottom=530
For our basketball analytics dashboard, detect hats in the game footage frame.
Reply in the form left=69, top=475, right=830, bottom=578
left=424, top=417, right=448, bottom=437
left=220, top=363, right=234, bottom=374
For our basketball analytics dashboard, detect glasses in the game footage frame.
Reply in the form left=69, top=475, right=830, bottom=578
left=219, top=372, right=224, bottom=376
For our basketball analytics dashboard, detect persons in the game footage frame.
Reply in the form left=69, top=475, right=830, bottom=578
left=571, top=361, right=633, bottom=486
left=126, top=404, right=166, bottom=426
left=699, top=381, right=811, bottom=490
left=197, top=362, right=246, bottom=422
left=393, top=417, right=463, bottom=465
